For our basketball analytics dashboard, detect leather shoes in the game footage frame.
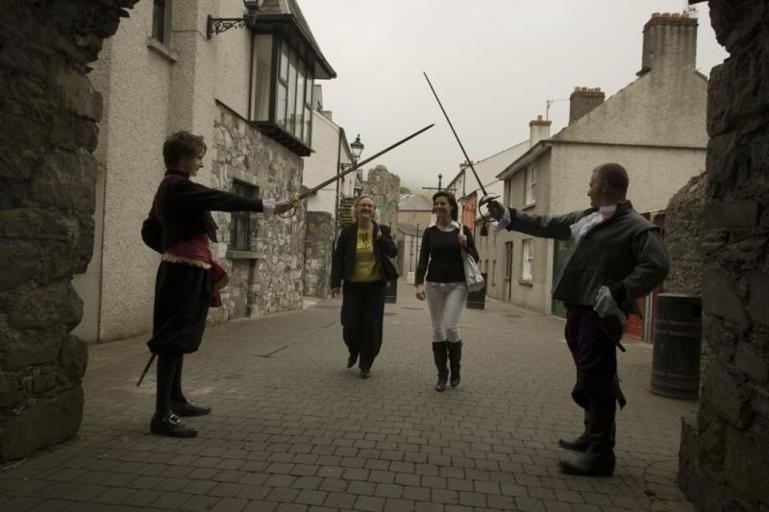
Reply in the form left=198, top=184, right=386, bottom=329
left=151, top=398, right=210, bottom=438
left=559, top=433, right=615, bottom=475
left=348, top=353, right=358, bottom=368
left=360, top=369, right=370, bottom=378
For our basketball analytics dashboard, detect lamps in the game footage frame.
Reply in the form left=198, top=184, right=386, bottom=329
left=339, top=133, right=366, bottom=171
left=204, top=1, right=266, bottom=43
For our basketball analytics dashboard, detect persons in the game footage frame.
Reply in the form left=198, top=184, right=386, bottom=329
left=330, top=194, right=400, bottom=380
left=415, top=192, right=479, bottom=392
left=141, top=130, right=294, bottom=438
left=488, top=164, right=669, bottom=476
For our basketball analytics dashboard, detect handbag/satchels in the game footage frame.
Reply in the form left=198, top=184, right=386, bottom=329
left=463, top=252, right=485, bottom=292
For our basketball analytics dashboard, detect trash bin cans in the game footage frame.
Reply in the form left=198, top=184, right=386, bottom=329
left=466, top=272, right=488, bottom=310
left=648, top=293, right=703, bottom=401
left=385, top=279, right=397, bottom=303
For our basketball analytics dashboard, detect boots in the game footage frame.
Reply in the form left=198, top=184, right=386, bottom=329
left=432, top=338, right=461, bottom=392
